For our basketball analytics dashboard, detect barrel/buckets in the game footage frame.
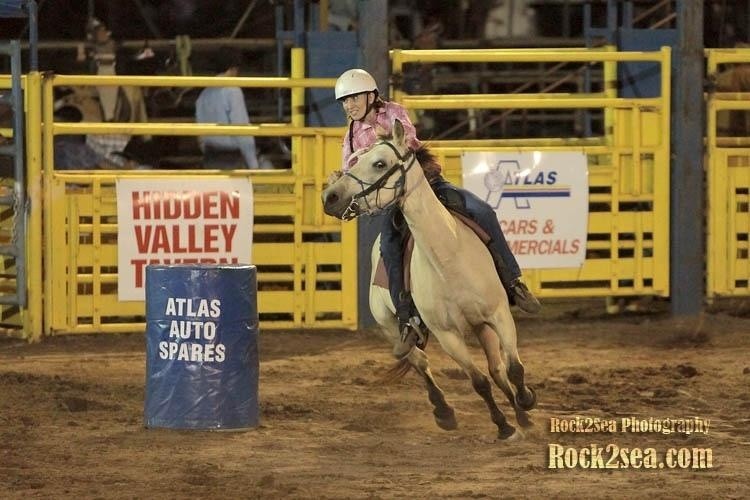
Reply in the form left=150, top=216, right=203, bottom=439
left=142, top=262, right=260, bottom=432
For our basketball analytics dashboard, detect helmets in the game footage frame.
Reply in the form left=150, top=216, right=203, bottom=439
left=335, top=69, right=379, bottom=100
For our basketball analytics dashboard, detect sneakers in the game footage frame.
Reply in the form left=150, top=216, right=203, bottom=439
left=395, top=327, right=424, bottom=359
left=509, top=281, right=541, bottom=314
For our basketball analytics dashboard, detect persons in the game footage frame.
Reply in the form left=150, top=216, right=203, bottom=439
left=18, top=0, right=537, bottom=170
left=328, top=68, right=541, bottom=360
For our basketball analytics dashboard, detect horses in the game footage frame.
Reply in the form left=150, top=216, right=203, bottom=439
left=320, top=117, right=540, bottom=447
